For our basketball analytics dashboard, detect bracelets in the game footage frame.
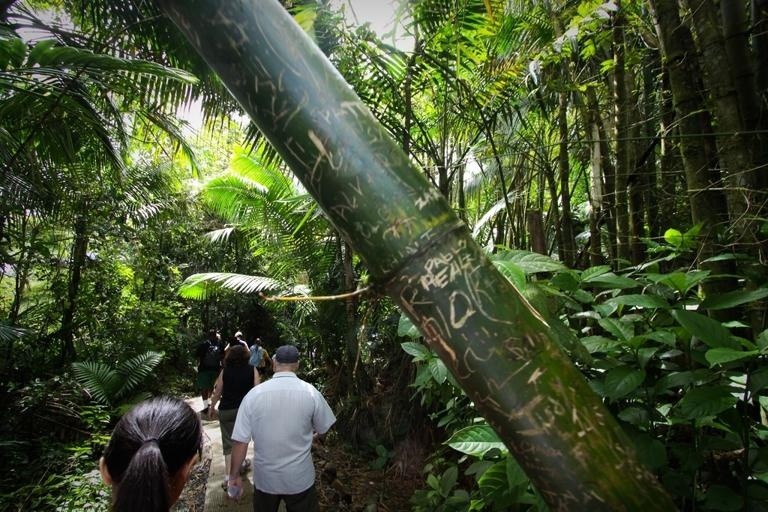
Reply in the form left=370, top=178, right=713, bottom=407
left=223, top=470, right=241, bottom=480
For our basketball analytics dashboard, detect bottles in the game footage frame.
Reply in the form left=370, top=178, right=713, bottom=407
left=226, top=484, right=247, bottom=500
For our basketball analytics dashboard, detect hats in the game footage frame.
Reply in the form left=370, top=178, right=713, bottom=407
left=276, top=346, right=299, bottom=363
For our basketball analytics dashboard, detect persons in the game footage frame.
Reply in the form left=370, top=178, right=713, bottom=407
left=208, top=345, right=260, bottom=492
left=98, top=394, right=203, bottom=512
left=194, top=327, right=279, bottom=420
left=226, top=343, right=339, bottom=512
